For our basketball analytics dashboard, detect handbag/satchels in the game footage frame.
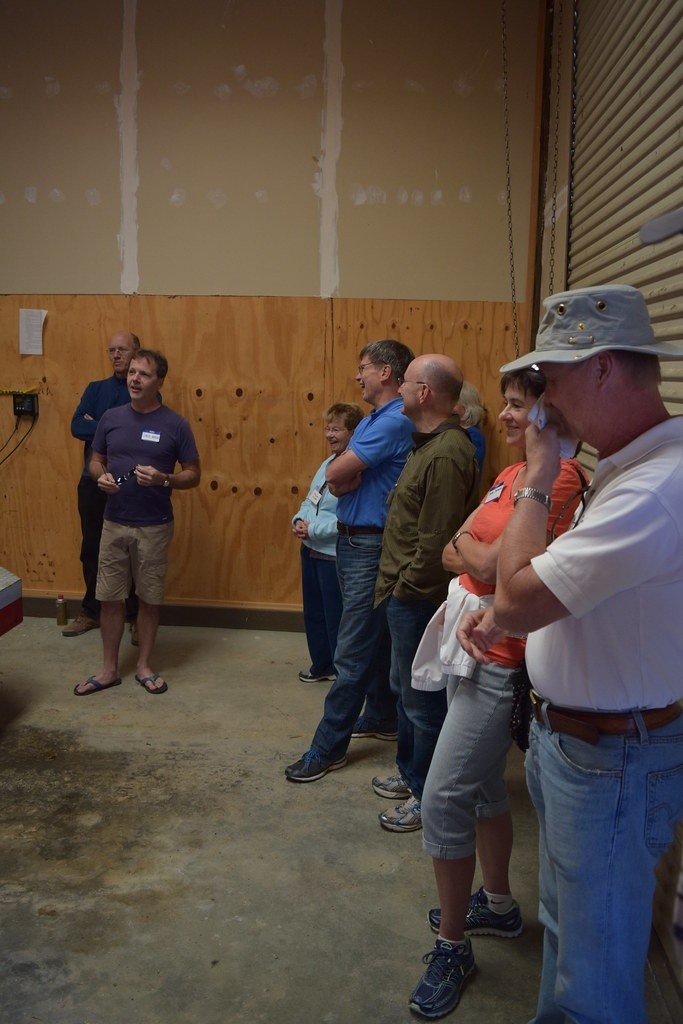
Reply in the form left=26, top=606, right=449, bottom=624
left=510, top=660, right=532, bottom=752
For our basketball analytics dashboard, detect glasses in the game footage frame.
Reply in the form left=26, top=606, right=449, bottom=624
left=106, top=348, right=134, bottom=354
left=101, top=450, right=137, bottom=485
left=324, top=426, right=352, bottom=435
left=397, top=377, right=432, bottom=393
left=551, top=486, right=590, bottom=542
left=357, top=361, right=387, bottom=374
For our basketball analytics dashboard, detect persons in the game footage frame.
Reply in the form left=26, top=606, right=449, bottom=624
left=284, top=340, right=415, bottom=781
left=493, top=285, right=683, bottom=1023
left=372, top=353, right=480, bottom=833
left=408, top=365, right=589, bottom=1017
left=291, top=402, right=364, bottom=682
left=73, top=350, right=201, bottom=696
left=454, top=380, right=485, bottom=467
left=62, top=332, right=141, bottom=646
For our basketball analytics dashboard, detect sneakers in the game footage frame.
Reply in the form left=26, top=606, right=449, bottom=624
left=427, top=887, right=522, bottom=937
left=299, top=668, right=337, bottom=682
left=130, top=622, right=138, bottom=645
left=351, top=716, right=398, bottom=741
left=62, top=614, right=100, bottom=636
left=372, top=774, right=410, bottom=798
left=409, top=936, right=475, bottom=1017
left=285, top=750, right=347, bottom=780
left=378, top=795, right=423, bottom=832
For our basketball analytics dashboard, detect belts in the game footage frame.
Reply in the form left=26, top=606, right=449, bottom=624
left=530, top=689, right=683, bottom=745
left=337, top=520, right=383, bottom=535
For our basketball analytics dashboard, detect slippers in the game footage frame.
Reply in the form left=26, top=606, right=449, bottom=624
left=74, top=676, right=121, bottom=696
left=135, top=673, right=167, bottom=693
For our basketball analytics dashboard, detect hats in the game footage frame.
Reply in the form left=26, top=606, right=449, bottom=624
left=499, top=284, right=683, bottom=373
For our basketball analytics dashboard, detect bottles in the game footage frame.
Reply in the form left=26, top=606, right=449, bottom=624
left=56, top=594, right=67, bottom=625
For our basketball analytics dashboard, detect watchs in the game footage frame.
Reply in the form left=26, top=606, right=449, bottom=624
left=163, top=473, right=170, bottom=487
left=452, top=531, right=474, bottom=549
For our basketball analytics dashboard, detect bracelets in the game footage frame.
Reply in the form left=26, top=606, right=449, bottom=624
left=514, top=489, right=551, bottom=513
